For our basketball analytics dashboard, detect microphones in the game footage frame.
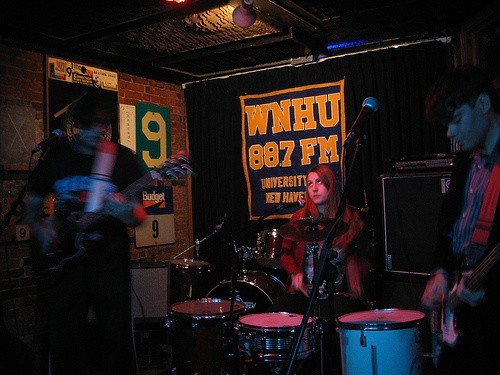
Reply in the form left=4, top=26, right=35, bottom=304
left=343, top=96, right=379, bottom=148
left=32, top=129, right=64, bottom=153
left=280, top=200, right=306, bottom=209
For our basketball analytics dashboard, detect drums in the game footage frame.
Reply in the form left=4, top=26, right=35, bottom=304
left=336, top=308, right=426, bottom=375
left=169, top=258, right=210, bottom=299
left=168, top=298, right=245, bottom=375
left=205, top=270, right=287, bottom=312
left=237, top=228, right=283, bottom=270
left=233, top=311, right=315, bottom=375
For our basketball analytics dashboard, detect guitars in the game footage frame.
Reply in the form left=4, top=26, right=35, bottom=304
left=430, top=242, right=500, bottom=368
left=31, top=154, right=194, bottom=271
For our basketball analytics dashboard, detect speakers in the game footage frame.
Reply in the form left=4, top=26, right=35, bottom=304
left=130, top=261, right=172, bottom=330
left=380, top=169, right=467, bottom=280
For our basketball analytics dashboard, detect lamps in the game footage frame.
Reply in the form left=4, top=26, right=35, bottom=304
left=233, top=0, right=256, bottom=28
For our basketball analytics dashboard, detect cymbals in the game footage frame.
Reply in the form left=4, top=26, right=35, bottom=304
left=277, top=216, right=350, bottom=243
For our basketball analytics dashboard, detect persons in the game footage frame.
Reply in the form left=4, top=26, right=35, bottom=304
left=420, top=64, right=500, bottom=375
left=267, top=165, right=367, bottom=314
left=26, top=91, right=146, bottom=375
